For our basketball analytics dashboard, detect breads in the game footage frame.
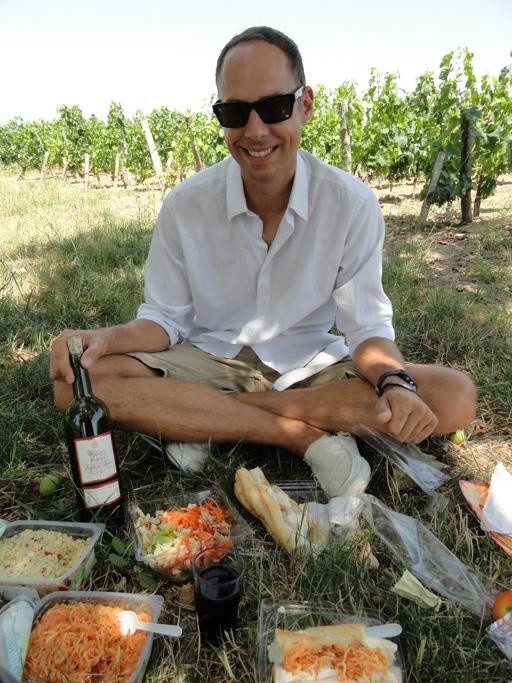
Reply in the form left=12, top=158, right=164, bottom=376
left=272, top=620, right=401, bottom=681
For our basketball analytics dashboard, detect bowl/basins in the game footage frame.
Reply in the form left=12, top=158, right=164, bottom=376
left=125, top=487, right=252, bottom=576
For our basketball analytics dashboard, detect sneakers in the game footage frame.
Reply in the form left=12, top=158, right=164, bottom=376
left=301, top=433, right=371, bottom=498
left=163, top=438, right=167, bottom=441
left=164, top=440, right=211, bottom=471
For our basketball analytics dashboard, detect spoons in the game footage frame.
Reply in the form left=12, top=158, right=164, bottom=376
left=116, top=611, right=183, bottom=638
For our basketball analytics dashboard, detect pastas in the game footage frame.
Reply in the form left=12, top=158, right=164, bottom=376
left=23, top=600, right=151, bottom=682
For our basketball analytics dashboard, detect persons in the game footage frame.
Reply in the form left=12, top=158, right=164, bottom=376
left=48, top=28, right=477, bottom=501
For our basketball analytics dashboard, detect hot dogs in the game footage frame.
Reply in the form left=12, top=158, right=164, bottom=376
left=231, top=463, right=328, bottom=564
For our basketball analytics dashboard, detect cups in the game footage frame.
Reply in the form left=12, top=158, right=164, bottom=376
left=191, top=547, right=245, bottom=649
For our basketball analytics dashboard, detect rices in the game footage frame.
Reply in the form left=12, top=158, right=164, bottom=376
left=1, top=528, right=94, bottom=595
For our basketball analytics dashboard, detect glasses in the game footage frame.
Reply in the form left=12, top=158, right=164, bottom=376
left=212, top=89, right=308, bottom=129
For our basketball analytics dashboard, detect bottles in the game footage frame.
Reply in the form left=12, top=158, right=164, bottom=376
left=64, top=334, right=126, bottom=529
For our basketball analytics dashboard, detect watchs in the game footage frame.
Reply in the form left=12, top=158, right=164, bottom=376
left=376, top=369, right=418, bottom=396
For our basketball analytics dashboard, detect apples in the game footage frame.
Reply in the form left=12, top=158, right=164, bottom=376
left=490, top=588, right=511, bottom=621
